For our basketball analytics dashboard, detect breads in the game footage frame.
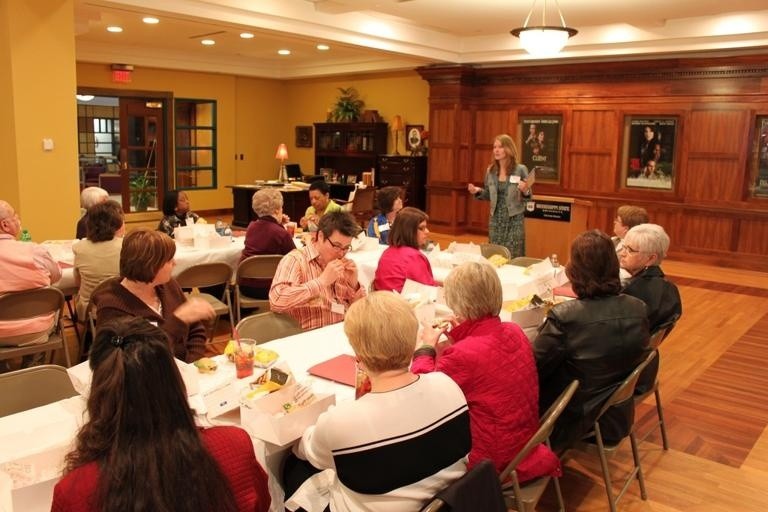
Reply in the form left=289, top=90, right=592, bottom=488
left=194, top=357, right=219, bottom=373
left=224, top=340, right=279, bottom=367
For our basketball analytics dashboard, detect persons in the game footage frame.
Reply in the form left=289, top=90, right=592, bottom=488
left=49, top=316, right=272, bottom=512
left=610, top=206, right=648, bottom=286
left=372, top=206, right=443, bottom=294
left=72, top=200, right=125, bottom=326
left=0, top=196, right=63, bottom=369
left=637, top=125, right=661, bottom=168
left=269, top=210, right=367, bottom=333
left=278, top=290, right=475, bottom=512
left=467, top=134, right=533, bottom=260
left=366, top=186, right=405, bottom=245
left=639, top=160, right=663, bottom=179
left=410, top=131, right=418, bottom=144
left=407, top=261, right=562, bottom=490
left=84, top=225, right=217, bottom=364
left=299, top=182, right=341, bottom=231
left=619, top=222, right=684, bottom=396
left=235, top=187, right=297, bottom=301
left=157, top=190, right=207, bottom=238
left=531, top=228, right=649, bottom=451
left=525, top=125, right=546, bottom=166
left=75, top=186, right=109, bottom=240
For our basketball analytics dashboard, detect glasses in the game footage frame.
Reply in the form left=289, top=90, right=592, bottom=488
left=621, top=244, right=639, bottom=256
left=326, top=236, right=352, bottom=253
left=418, top=226, right=426, bottom=232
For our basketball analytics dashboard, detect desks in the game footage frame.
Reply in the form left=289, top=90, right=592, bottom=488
left=224, top=180, right=312, bottom=232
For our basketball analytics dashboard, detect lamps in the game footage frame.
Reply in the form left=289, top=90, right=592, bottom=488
left=391, top=115, right=403, bottom=155
left=274, top=143, right=288, bottom=183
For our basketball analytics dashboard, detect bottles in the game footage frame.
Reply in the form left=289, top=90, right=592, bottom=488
left=223, top=224, right=233, bottom=242
left=20, top=229, right=32, bottom=244
left=216, top=218, right=223, bottom=236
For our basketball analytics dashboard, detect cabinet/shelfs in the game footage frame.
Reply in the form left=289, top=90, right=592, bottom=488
left=378, top=155, right=428, bottom=211
left=314, top=122, right=389, bottom=201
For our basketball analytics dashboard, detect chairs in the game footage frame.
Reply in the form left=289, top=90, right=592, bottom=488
left=332, top=186, right=377, bottom=230
left=285, top=163, right=302, bottom=178
left=0, top=285, right=72, bottom=367
left=306, top=175, right=324, bottom=183
left=509, top=257, right=543, bottom=271
left=175, top=261, right=235, bottom=343
left=233, top=254, right=285, bottom=323
left=571, top=349, right=658, bottom=512
left=631, top=311, right=683, bottom=480
left=498, top=379, right=580, bottom=512
left=480, top=244, right=511, bottom=264
left=421, top=458, right=498, bottom=512
left=232, top=311, right=302, bottom=349
left=0, top=365, right=82, bottom=418
left=73, top=276, right=123, bottom=363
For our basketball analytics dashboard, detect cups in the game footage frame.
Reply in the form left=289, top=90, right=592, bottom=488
left=229, top=341, right=258, bottom=382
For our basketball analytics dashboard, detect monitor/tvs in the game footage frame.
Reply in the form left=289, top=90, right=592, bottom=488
left=285, top=164, right=303, bottom=183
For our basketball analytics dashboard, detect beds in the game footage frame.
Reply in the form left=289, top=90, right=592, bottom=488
left=508, top=1, right=578, bottom=58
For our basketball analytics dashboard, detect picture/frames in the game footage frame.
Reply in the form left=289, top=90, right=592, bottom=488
left=406, top=124, right=425, bottom=151
left=623, top=116, right=679, bottom=191
left=295, top=125, right=313, bottom=147
left=749, top=118, right=768, bottom=197
left=518, top=115, right=563, bottom=183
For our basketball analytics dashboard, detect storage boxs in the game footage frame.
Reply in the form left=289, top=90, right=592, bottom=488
left=500, top=297, right=551, bottom=330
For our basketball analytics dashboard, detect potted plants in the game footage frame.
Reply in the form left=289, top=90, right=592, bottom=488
left=128, top=175, right=156, bottom=211
left=326, top=87, right=365, bottom=123
